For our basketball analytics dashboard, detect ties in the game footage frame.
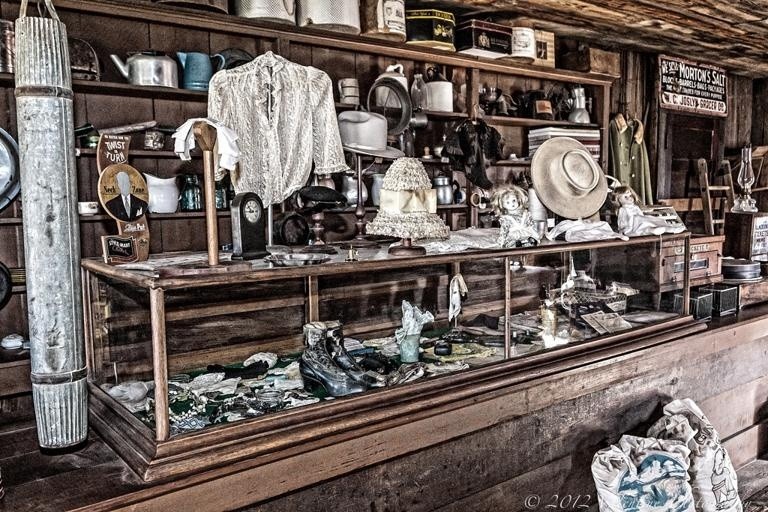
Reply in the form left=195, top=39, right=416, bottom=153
left=124, top=195, right=131, bottom=218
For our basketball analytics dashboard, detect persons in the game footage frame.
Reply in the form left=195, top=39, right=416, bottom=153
left=104, top=171, right=149, bottom=221
left=488, top=183, right=540, bottom=249
left=608, top=185, right=685, bottom=237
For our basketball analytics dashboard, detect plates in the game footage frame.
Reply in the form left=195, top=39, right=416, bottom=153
left=0, top=128, right=22, bottom=209
left=262, top=253, right=330, bottom=265
left=724, top=277, right=762, bottom=284
left=0, top=260, right=13, bottom=310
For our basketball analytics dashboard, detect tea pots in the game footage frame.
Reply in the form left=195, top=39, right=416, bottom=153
left=143, top=172, right=181, bottom=214
left=107, top=51, right=227, bottom=94
left=429, top=171, right=459, bottom=205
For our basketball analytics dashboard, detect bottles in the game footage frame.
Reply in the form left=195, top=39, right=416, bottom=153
left=183, top=173, right=227, bottom=211
left=370, top=173, right=383, bottom=207
left=411, top=73, right=427, bottom=109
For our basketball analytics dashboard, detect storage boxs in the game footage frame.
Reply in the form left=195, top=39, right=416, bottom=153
left=625, top=233, right=726, bottom=296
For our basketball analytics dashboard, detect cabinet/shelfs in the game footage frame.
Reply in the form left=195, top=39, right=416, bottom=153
left=0, top=0, right=707, bottom=484
left=722, top=211, right=768, bottom=268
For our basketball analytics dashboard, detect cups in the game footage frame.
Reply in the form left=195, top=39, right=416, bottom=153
left=398, top=332, right=421, bottom=363
left=76, top=201, right=98, bottom=217
left=532, top=219, right=546, bottom=240
left=336, top=78, right=359, bottom=105
left=428, top=81, right=454, bottom=112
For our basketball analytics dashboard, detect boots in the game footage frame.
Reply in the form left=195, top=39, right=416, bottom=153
left=298, top=319, right=387, bottom=397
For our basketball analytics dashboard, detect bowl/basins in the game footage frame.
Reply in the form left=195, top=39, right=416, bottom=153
left=722, top=258, right=761, bottom=276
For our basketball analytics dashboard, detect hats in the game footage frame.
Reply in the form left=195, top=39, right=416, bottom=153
left=529, top=137, right=609, bottom=219
left=337, top=109, right=405, bottom=160
